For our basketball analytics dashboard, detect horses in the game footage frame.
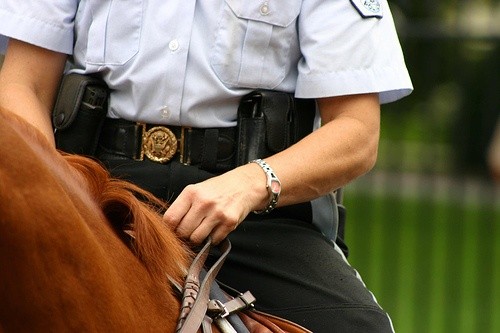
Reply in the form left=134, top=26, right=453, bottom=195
left=0, top=105, right=313, bottom=333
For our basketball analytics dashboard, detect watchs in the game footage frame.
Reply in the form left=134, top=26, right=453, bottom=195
left=249, top=158, right=282, bottom=217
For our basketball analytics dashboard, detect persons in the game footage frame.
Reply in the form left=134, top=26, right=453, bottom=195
left=0, top=0, right=416, bottom=333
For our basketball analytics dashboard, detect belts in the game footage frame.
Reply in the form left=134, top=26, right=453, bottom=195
left=56, top=124, right=237, bottom=169
